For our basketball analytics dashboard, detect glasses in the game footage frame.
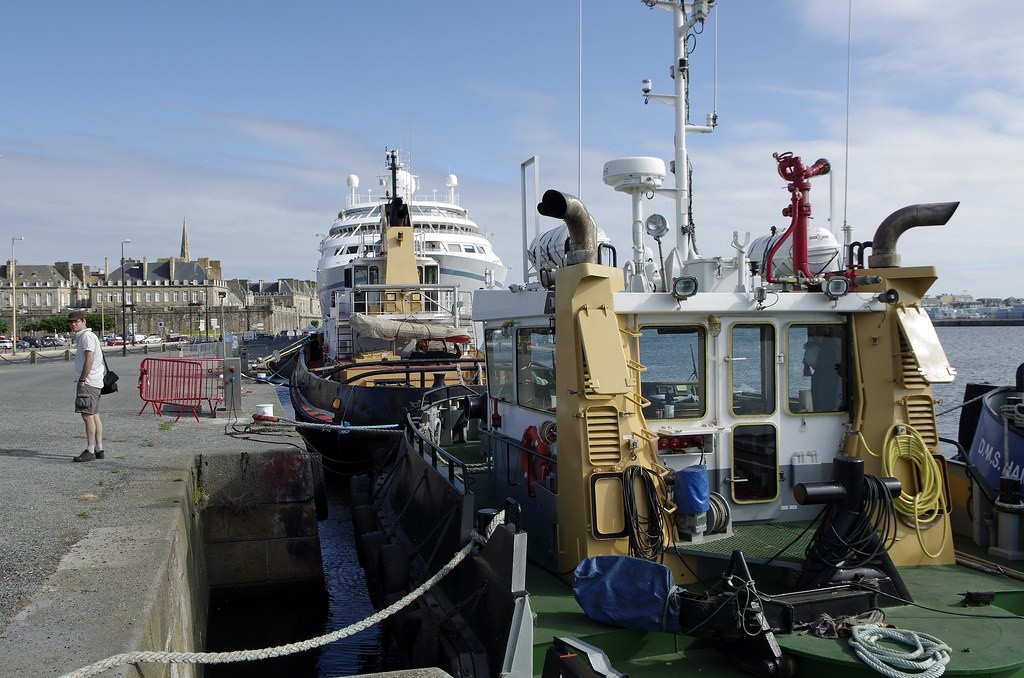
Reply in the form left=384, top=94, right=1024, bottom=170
left=69, top=320, right=80, bottom=324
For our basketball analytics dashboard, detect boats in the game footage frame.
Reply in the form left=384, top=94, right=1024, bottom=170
left=360, top=2, right=1022, bottom=678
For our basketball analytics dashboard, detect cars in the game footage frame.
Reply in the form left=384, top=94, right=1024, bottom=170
left=1, top=332, right=189, bottom=349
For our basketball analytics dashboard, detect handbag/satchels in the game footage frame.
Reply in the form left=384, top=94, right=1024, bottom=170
left=101, top=371, right=119, bottom=394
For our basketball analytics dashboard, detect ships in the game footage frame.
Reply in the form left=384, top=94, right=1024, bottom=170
left=288, top=148, right=508, bottom=464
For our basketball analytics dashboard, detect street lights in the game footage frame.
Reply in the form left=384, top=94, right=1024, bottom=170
left=11, top=237, right=24, bottom=357
left=204, top=266, right=213, bottom=343
left=121, top=238, right=132, bottom=357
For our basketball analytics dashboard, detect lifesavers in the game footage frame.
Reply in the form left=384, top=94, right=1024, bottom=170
left=521, top=426, right=551, bottom=493
left=322, top=343, right=329, bottom=361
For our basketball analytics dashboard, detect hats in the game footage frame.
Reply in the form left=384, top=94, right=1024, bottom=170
left=66, top=311, right=83, bottom=322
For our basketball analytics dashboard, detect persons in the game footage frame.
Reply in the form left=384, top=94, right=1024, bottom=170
left=68, top=311, right=105, bottom=462
left=219, top=335, right=223, bottom=342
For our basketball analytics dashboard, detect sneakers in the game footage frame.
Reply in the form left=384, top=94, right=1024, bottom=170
left=94, top=450, right=104, bottom=459
left=73, top=449, right=96, bottom=462
